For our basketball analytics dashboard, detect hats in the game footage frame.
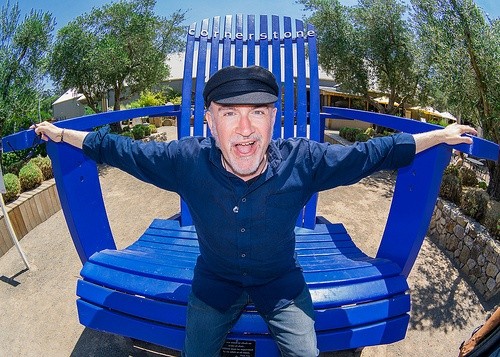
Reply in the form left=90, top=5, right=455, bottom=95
left=203, top=65, right=279, bottom=107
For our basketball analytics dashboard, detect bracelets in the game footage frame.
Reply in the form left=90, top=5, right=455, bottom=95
left=61, top=127, right=64, bottom=143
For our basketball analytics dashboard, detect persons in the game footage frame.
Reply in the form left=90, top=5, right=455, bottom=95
left=25, top=64, right=477, bottom=356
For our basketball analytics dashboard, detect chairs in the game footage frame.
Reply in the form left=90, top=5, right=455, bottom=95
left=1, top=13, right=499, bottom=357
